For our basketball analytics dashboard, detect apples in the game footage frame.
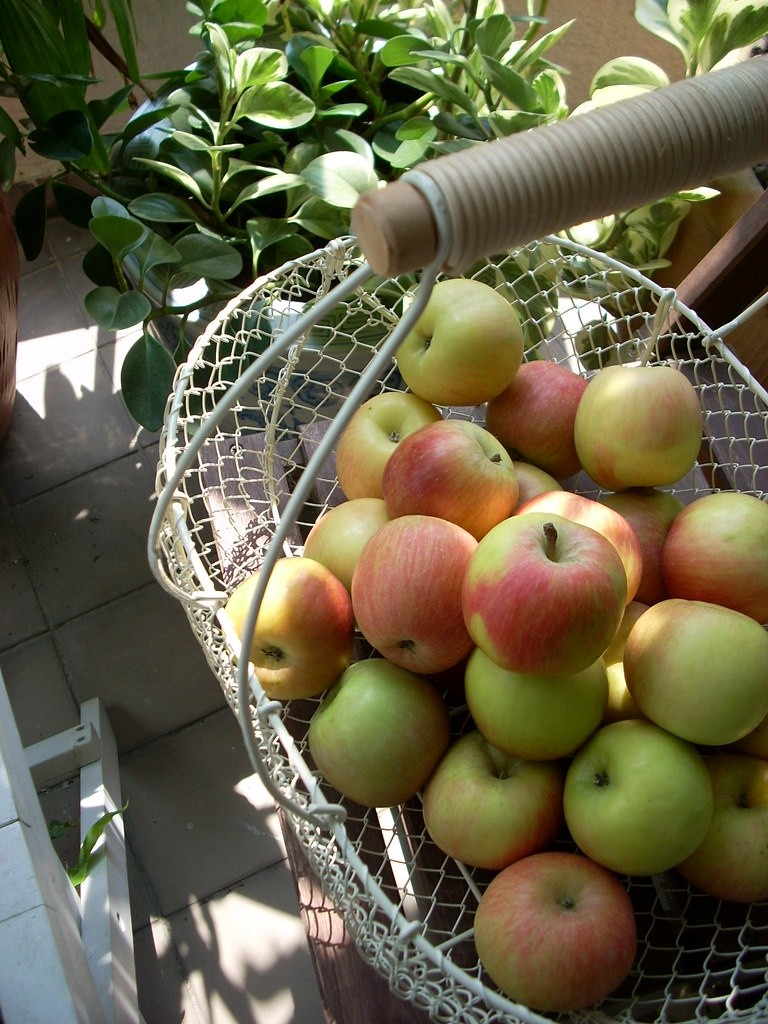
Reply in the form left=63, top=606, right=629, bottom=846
left=224, top=278, right=768, bottom=1015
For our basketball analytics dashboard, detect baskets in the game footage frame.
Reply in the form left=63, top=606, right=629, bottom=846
left=147, top=169, right=768, bottom=1024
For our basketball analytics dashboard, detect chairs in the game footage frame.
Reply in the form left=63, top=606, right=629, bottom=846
left=276, top=188, right=767, bottom=1024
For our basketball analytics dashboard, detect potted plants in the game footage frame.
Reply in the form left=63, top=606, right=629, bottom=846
left=0, top=0, right=144, bottom=443
left=632, top=0, right=768, bottom=340
left=17, top=0, right=623, bottom=438
